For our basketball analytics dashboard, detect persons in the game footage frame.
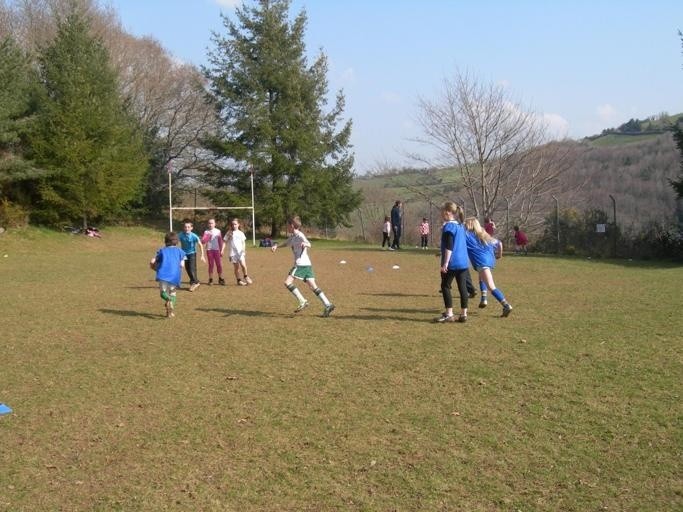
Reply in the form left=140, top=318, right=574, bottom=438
left=270, top=215, right=335, bottom=318
left=437, top=266, right=478, bottom=299
left=199, top=217, right=227, bottom=287
left=380, top=214, right=395, bottom=251
left=463, top=216, right=513, bottom=319
left=389, top=199, right=402, bottom=251
left=219, top=216, right=252, bottom=286
left=176, top=218, right=206, bottom=293
left=483, top=214, right=495, bottom=236
left=418, top=216, right=430, bottom=251
left=148, top=229, right=186, bottom=319
left=512, top=224, right=528, bottom=256
left=432, top=201, right=469, bottom=323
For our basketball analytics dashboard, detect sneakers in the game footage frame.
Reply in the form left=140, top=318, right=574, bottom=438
left=208, top=278, right=226, bottom=285
left=236, top=276, right=253, bottom=285
left=189, top=282, right=200, bottom=292
left=164, top=296, right=176, bottom=319
left=291, top=300, right=309, bottom=313
left=379, top=244, right=430, bottom=252
left=320, top=303, right=337, bottom=317
left=432, top=287, right=515, bottom=323
